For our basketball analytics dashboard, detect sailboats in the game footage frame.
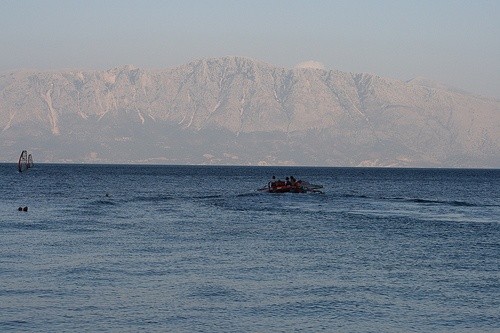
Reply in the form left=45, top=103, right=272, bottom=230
left=27, top=153, right=35, bottom=169
left=19, top=150, right=27, bottom=172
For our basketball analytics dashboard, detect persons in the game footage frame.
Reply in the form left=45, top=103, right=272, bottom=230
left=271, top=175, right=277, bottom=184
left=285, top=176, right=290, bottom=185
left=290, top=175, right=301, bottom=186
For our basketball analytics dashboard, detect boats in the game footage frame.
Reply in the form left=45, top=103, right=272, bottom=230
left=248, top=180, right=324, bottom=193
